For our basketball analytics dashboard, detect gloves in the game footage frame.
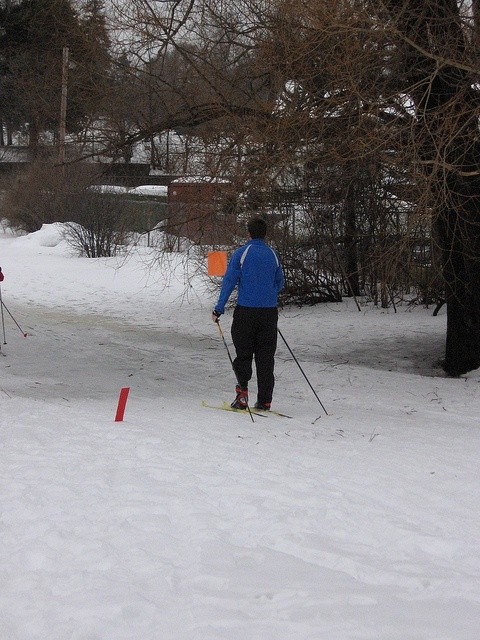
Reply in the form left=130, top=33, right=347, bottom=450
left=211, top=310, right=221, bottom=322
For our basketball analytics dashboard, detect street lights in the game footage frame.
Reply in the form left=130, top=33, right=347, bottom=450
left=59, top=61, right=80, bottom=164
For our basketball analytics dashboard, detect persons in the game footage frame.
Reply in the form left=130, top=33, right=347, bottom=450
left=211, top=217, right=288, bottom=414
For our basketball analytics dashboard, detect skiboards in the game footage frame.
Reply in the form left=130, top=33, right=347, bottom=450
left=200, top=400, right=293, bottom=419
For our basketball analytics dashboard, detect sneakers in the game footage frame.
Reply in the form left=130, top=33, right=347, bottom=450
left=230, top=394, right=249, bottom=410
left=254, top=402, right=271, bottom=411
